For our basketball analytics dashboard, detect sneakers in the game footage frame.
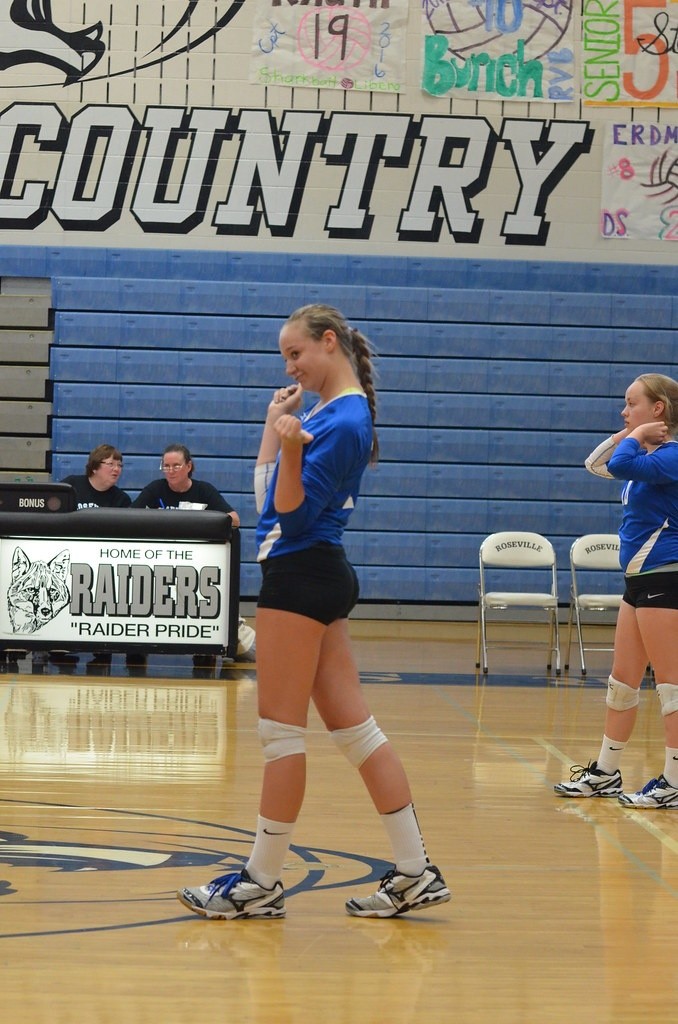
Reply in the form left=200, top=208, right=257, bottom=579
left=553, top=760, right=624, bottom=797
left=618, top=773, right=678, bottom=809
left=346, top=864, right=453, bottom=918
left=178, top=867, right=287, bottom=918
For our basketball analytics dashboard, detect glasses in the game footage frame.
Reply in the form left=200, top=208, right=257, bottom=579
left=159, top=461, right=187, bottom=472
left=99, top=460, right=123, bottom=468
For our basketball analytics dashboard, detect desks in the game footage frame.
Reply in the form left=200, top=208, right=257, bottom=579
left=0, top=506, right=242, bottom=668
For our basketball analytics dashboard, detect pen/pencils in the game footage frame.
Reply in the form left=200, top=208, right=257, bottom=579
left=159, top=498, right=166, bottom=510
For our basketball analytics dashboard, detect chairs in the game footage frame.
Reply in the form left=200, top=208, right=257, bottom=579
left=474, top=532, right=566, bottom=678
left=565, top=534, right=651, bottom=678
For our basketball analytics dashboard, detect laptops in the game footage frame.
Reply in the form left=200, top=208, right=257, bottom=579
left=0, top=483, right=75, bottom=513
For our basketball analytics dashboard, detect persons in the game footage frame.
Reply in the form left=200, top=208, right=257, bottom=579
left=176, top=304, right=452, bottom=923
left=129, top=445, right=240, bottom=529
left=56, top=444, right=131, bottom=511
left=553, top=373, right=678, bottom=812
